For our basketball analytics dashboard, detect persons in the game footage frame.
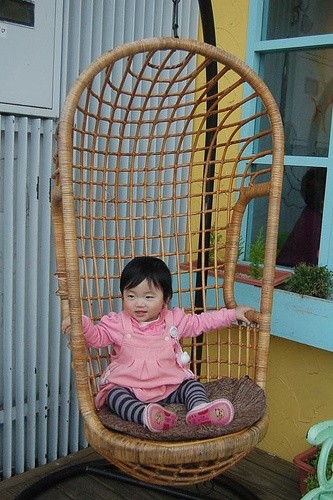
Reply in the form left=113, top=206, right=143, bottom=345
left=61, top=256, right=255, bottom=433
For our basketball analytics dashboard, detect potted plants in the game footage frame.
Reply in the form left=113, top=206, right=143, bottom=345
left=179, top=222, right=293, bottom=287
left=292, top=446, right=333, bottom=497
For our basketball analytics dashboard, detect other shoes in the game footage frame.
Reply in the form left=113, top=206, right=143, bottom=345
left=185, top=398, right=234, bottom=427
left=142, top=402, right=177, bottom=434
